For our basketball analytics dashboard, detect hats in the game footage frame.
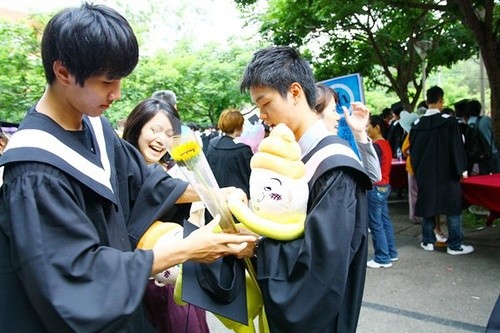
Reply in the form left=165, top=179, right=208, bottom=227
left=180, top=218, right=250, bottom=327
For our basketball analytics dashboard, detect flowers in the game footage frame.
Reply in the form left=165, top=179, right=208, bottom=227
left=171, top=141, right=263, bottom=296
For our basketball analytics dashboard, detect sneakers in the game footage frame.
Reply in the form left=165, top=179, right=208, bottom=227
left=421, top=242, right=434, bottom=252
left=366, top=259, right=393, bottom=268
left=390, top=258, right=399, bottom=261
left=446, top=245, right=474, bottom=255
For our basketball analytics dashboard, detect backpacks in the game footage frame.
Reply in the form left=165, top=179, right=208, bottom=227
left=465, top=116, right=492, bottom=164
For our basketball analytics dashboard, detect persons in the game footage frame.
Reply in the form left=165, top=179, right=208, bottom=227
left=0, top=1, right=256, bottom=333
left=227, top=46, right=373, bottom=333
left=0, top=87, right=500, bottom=333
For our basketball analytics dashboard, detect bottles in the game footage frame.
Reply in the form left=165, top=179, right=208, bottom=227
left=396, top=147, right=402, bottom=160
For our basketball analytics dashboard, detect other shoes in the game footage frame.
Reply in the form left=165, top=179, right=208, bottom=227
left=436, top=232, right=447, bottom=243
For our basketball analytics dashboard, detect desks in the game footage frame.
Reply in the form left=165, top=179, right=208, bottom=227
left=387, top=158, right=409, bottom=202
left=461, top=173, right=500, bottom=212
